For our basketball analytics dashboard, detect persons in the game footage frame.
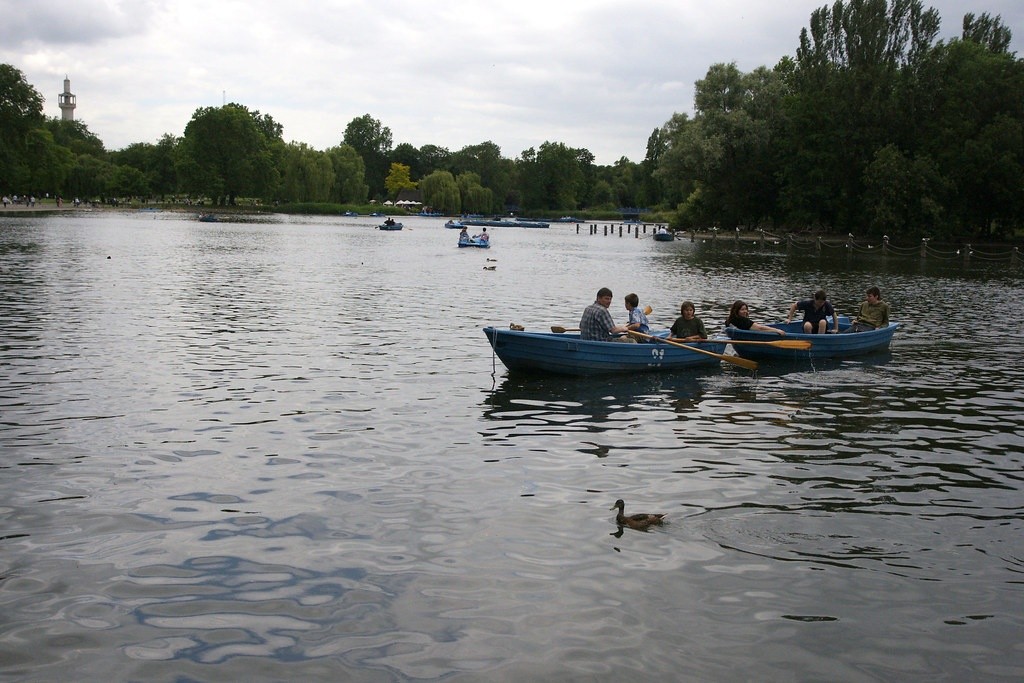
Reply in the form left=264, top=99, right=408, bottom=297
left=666, top=301, right=707, bottom=342
left=624, top=293, right=649, bottom=334
left=784, top=290, right=838, bottom=334
left=459, top=226, right=489, bottom=242
left=660, top=227, right=666, bottom=234
left=579, top=287, right=637, bottom=343
left=843, top=286, right=890, bottom=333
left=384, top=217, right=395, bottom=226
left=3, top=193, right=79, bottom=208
left=727, top=300, right=786, bottom=337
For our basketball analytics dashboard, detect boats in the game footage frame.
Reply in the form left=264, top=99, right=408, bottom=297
left=379, top=222, right=402, bottom=230
left=445, top=220, right=466, bottom=229
left=725, top=314, right=899, bottom=360
left=457, top=235, right=489, bottom=249
left=343, top=209, right=358, bottom=217
left=652, top=231, right=675, bottom=242
left=370, top=212, right=378, bottom=217
left=482, top=323, right=732, bottom=376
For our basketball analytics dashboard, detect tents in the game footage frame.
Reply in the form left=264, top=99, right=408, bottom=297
left=383, top=200, right=423, bottom=208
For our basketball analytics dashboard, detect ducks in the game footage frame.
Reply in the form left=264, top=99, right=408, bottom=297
left=609, top=499, right=668, bottom=528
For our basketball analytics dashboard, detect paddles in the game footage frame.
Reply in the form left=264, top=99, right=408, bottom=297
left=626, top=305, right=653, bottom=327
left=675, top=236, right=681, bottom=240
left=551, top=325, right=581, bottom=334
left=668, top=338, right=813, bottom=350
left=639, top=234, right=653, bottom=240
left=627, top=329, right=758, bottom=370
